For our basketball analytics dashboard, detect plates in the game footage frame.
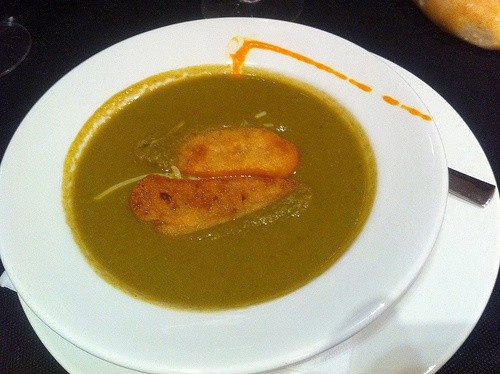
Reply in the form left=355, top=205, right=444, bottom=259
left=17, top=49, right=500, bottom=374
left=0, top=20, right=31, bottom=77
left=0, top=17, right=448, bottom=374
